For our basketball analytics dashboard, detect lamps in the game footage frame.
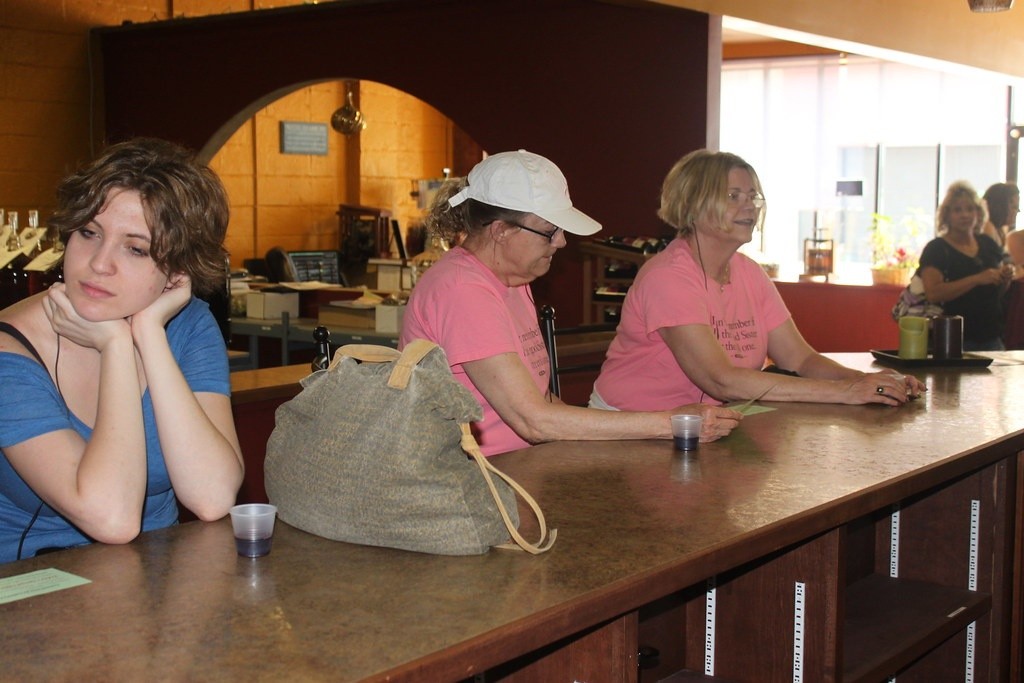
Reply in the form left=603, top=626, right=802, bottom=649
left=330, top=81, right=367, bottom=139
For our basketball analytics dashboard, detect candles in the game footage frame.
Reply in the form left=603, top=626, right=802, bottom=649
left=932, top=313, right=963, bottom=354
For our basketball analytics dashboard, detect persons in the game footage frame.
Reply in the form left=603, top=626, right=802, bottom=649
left=916, top=180, right=1024, bottom=352
left=396, top=149, right=742, bottom=460
left=588, top=149, right=927, bottom=412
left=0, top=138, right=246, bottom=560
left=253, top=247, right=308, bottom=315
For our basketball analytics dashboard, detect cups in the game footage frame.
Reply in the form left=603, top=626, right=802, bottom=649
left=229, top=504, right=277, bottom=558
left=899, top=317, right=928, bottom=360
left=934, top=315, right=964, bottom=358
left=671, top=414, right=703, bottom=451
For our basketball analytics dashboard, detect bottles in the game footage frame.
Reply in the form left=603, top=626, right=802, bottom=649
left=594, top=235, right=668, bottom=322
left=23, top=210, right=46, bottom=299
left=44, top=224, right=64, bottom=289
left=4, top=211, right=29, bottom=310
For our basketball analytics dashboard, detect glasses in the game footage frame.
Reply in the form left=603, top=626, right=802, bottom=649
left=482, top=218, right=562, bottom=244
left=728, top=192, right=765, bottom=208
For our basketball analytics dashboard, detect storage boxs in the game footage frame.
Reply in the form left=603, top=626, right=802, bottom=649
left=374, top=304, right=407, bottom=335
left=247, top=291, right=299, bottom=321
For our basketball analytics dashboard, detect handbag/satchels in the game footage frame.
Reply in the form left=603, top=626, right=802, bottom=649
left=264, top=340, right=557, bottom=557
left=892, top=240, right=951, bottom=324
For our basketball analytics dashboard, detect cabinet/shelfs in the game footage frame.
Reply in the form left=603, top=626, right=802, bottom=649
left=577, top=240, right=656, bottom=327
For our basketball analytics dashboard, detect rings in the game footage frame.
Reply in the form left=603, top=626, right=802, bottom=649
left=877, top=387, right=884, bottom=395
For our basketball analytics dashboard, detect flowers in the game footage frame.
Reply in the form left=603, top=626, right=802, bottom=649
left=866, top=212, right=919, bottom=268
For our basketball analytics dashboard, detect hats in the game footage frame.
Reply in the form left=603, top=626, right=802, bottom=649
left=447, top=148, right=602, bottom=236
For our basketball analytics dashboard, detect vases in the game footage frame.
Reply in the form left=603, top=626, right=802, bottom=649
left=871, top=268, right=911, bottom=286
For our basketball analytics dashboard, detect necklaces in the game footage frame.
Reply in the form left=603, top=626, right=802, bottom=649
left=703, top=264, right=729, bottom=293
